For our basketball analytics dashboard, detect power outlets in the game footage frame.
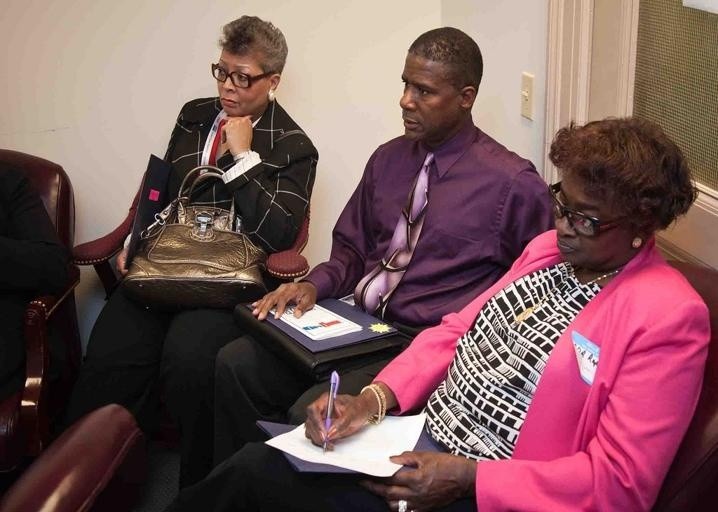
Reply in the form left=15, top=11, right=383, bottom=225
left=520, top=72, right=534, bottom=121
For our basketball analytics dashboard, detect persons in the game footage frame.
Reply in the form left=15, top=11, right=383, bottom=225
left=211, top=27, right=558, bottom=471
left=162, top=117, right=713, bottom=512
left=85, top=14, right=319, bottom=497
left=0, top=158, right=69, bottom=408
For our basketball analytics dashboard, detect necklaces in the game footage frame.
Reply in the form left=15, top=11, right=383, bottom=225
left=514, top=266, right=626, bottom=325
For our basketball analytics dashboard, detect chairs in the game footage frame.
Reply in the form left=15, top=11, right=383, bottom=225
left=1, top=401, right=142, bottom=512
left=651, top=258, right=718, bottom=511
left=0, top=149, right=81, bottom=456
left=74, top=169, right=309, bottom=300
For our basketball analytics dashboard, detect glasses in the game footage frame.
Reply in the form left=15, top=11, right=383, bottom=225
left=211, top=63, right=266, bottom=88
left=548, top=183, right=626, bottom=238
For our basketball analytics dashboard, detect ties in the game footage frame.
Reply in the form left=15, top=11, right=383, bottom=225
left=354, top=151, right=436, bottom=319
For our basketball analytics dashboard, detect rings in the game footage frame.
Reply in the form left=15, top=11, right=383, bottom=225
left=398, top=499, right=408, bottom=512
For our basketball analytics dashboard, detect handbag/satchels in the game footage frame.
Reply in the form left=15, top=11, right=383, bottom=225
left=121, top=201, right=267, bottom=308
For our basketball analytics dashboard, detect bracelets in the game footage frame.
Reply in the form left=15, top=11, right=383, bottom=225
left=360, top=383, right=387, bottom=425
left=233, top=150, right=252, bottom=162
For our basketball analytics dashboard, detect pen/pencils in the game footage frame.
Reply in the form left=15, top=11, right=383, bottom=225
left=323, top=370, right=340, bottom=455
